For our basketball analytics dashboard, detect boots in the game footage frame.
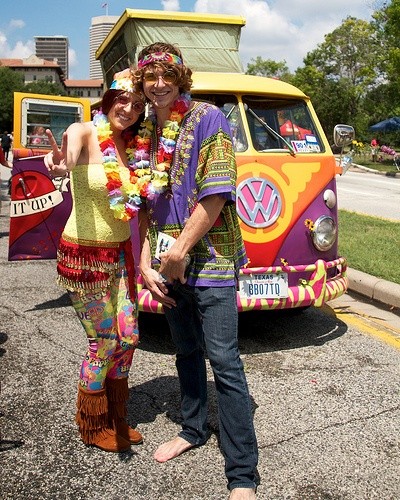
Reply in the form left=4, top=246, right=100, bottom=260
left=105, top=375, right=143, bottom=445
left=76, top=383, right=131, bottom=452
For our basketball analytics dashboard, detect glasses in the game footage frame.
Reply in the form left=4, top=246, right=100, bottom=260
left=113, top=95, right=145, bottom=115
left=143, top=70, right=176, bottom=84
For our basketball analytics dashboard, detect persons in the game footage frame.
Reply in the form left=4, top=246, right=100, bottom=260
left=370, top=136, right=380, bottom=163
left=30, top=126, right=44, bottom=144
left=43, top=42, right=260, bottom=500
left=1, top=131, right=14, bottom=161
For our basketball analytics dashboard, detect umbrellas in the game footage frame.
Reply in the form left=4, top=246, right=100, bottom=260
left=368, top=117, right=400, bottom=131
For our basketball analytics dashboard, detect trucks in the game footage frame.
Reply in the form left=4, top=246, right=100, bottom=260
left=8, top=8, right=356, bottom=313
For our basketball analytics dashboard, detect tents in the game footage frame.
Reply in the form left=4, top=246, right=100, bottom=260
left=280, top=120, right=312, bottom=141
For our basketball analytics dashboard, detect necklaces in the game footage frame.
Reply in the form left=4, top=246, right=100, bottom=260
left=132, top=93, right=193, bottom=202
left=92, top=109, right=142, bottom=222
